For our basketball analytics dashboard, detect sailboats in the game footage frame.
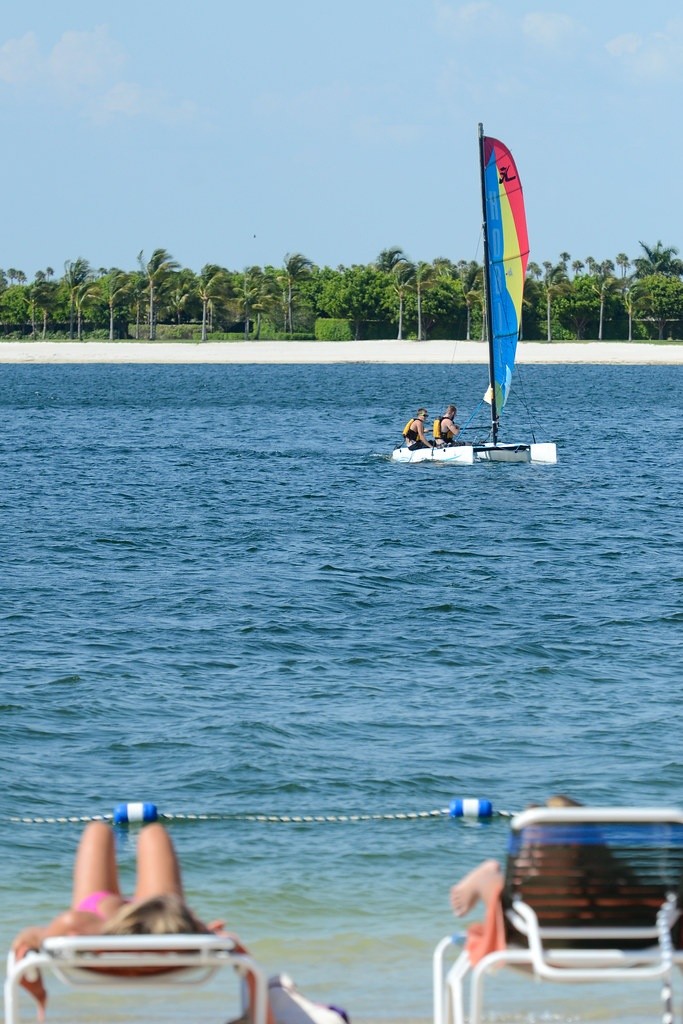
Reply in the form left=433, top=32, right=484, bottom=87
left=393, top=123, right=559, bottom=464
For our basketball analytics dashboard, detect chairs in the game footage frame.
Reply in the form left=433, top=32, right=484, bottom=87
left=432, top=805, right=683, bottom=1024
left=2, top=936, right=269, bottom=1023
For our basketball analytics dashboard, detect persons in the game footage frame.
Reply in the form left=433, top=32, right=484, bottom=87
left=402, top=408, right=436, bottom=451
left=450, top=794, right=643, bottom=969
left=11, top=820, right=214, bottom=975
left=433, top=406, right=460, bottom=446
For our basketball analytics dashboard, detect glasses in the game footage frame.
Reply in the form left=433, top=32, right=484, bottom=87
left=419, top=414, right=429, bottom=417
